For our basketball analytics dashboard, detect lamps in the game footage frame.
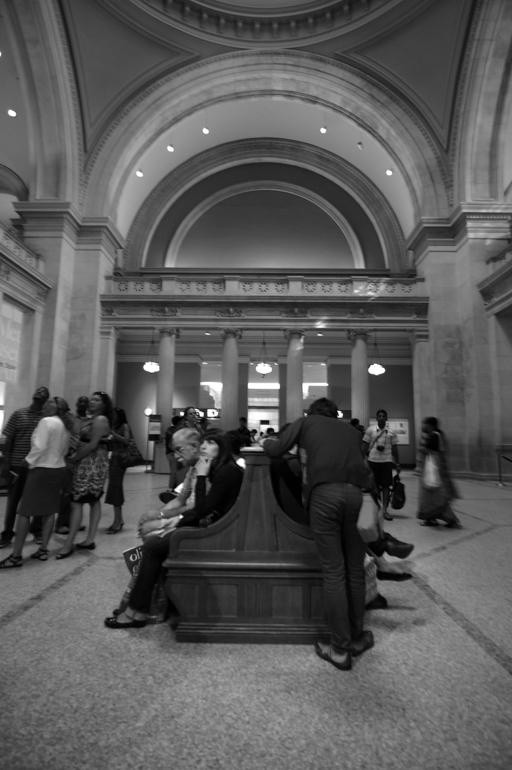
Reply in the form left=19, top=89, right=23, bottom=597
left=255, top=331, right=274, bottom=378
left=143, top=329, right=161, bottom=374
left=366, top=331, right=386, bottom=378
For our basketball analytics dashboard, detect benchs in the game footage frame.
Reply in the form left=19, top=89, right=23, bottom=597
left=161, top=448, right=335, bottom=645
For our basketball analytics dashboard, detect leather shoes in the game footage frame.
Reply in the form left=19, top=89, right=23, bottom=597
left=75, top=542, right=95, bottom=550
left=56, top=547, right=74, bottom=559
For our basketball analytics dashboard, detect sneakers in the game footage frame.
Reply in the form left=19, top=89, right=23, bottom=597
left=383, top=510, right=393, bottom=520
left=33, top=535, right=42, bottom=544
left=103, top=607, right=146, bottom=627
left=384, top=531, right=413, bottom=559
left=376, top=568, right=412, bottom=581
left=314, top=637, right=352, bottom=670
left=351, top=631, right=373, bottom=655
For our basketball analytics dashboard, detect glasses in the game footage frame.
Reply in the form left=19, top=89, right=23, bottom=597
left=177, top=444, right=187, bottom=453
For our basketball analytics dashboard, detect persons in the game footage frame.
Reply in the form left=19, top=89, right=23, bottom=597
left=239, top=417, right=251, bottom=439
left=136, top=428, right=212, bottom=543
left=103, top=427, right=245, bottom=629
left=257, top=432, right=264, bottom=442
left=278, top=421, right=414, bottom=559
left=164, top=416, right=180, bottom=489
left=361, top=408, right=401, bottom=521
left=257, top=397, right=374, bottom=670
left=55, top=390, right=113, bottom=560
left=225, top=431, right=246, bottom=469
left=0, top=386, right=80, bottom=547
left=103, top=407, right=137, bottom=535
left=250, top=429, right=257, bottom=442
left=180, top=406, right=199, bottom=432
left=416, top=416, right=464, bottom=529
left=367, top=549, right=414, bottom=581
left=70, top=396, right=89, bottom=437
left=267, top=428, right=274, bottom=435
left=0, top=396, right=73, bottom=569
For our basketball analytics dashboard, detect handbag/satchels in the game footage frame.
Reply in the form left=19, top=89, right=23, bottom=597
left=355, top=489, right=385, bottom=557
left=118, top=446, right=144, bottom=468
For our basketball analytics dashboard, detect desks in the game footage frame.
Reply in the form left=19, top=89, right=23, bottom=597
left=495, top=440, right=512, bottom=487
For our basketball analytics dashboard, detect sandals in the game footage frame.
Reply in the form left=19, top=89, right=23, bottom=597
left=0, top=554, right=23, bottom=569
left=30, top=548, right=48, bottom=560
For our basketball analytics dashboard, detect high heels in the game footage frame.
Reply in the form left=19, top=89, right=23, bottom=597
left=106, top=525, right=122, bottom=535
left=104, top=521, right=124, bottom=530
